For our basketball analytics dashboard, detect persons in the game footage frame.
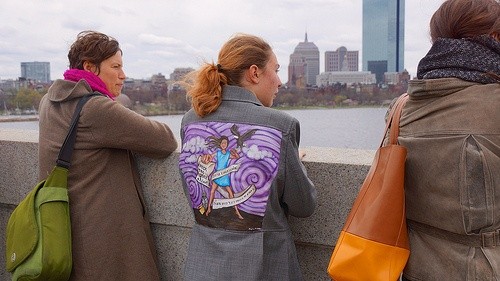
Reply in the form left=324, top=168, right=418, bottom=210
left=179, top=32, right=318, bottom=281
left=38, top=29, right=178, bottom=280
left=382, top=0, right=500, bottom=281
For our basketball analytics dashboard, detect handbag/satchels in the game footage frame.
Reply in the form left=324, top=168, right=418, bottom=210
left=327, top=92, right=410, bottom=281
left=6, top=92, right=107, bottom=281
